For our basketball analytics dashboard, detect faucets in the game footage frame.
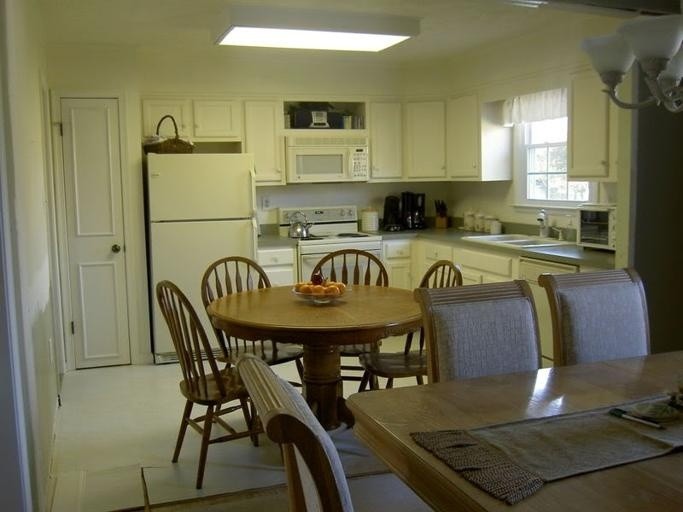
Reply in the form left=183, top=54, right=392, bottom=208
left=537, top=209, right=549, bottom=238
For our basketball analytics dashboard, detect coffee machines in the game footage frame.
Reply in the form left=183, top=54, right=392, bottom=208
left=400, top=191, right=428, bottom=230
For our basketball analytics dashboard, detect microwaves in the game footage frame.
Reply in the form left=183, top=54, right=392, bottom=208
left=285, top=135, right=369, bottom=184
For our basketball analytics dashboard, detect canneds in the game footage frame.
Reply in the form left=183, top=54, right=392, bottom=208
left=464, top=212, right=474, bottom=231
left=474, top=216, right=485, bottom=232
left=484, top=217, right=495, bottom=232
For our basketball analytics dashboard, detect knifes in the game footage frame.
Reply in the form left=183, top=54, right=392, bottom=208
left=433, top=198, right=446, bottom=216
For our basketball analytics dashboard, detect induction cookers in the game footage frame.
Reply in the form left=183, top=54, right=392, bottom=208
left=279, top=233, right=382, bottom=244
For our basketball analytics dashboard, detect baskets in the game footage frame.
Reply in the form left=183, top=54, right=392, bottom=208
left=144, top=115, right=195, bottom=153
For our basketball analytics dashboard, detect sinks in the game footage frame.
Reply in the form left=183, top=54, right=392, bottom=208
left=470, top=233, right=531, bottom=242
left=503, top=238, right=555, bottom=247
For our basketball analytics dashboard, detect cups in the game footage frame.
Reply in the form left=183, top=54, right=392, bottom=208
left=460, top=210, right=502, bottom=235
left=341, top=115, right=352, bottom=130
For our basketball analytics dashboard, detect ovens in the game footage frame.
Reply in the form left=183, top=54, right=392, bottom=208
left=574, top=202, right=616, bottom=252
left=298, top=250, right=382, bottom=284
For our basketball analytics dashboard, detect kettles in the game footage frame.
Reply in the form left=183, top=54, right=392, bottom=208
left=382, top=195, right=401, bottom=232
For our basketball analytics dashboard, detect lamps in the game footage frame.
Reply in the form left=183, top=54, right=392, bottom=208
left=208, top=5, right=422, bottom=53
left=583, top=19, right=682, bottom=112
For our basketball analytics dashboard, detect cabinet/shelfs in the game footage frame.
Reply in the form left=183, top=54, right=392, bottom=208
left=518, top=258, right=579, bottom=362
left=61, top=99, right=133, bottom=370
left=383, top=240, right=411, bottom=287
left=445, top=93, right=513, bottom=180
left=569, top=69, right=610, bottom=179
left=424, top=242, right=451, bottom=271
left=253, top=102, right=403, bottom=187
left=257, top=247, right=297, bottom=285
left=454, top=250, right=515, bottom=285
left=142, top=99, right=241, bottom=138
left=405, top=101, right=447, bottom=179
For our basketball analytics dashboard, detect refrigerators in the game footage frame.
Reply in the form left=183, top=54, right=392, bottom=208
left=146, top=152, right=261, bottom=365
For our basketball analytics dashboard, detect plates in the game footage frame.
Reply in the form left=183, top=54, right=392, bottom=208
left=291, top=287, right=353, bottom=304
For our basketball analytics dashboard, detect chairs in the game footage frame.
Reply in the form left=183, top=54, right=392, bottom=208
left=155, top=279, right=261, bottom=492
left=202, top=256, right=303, bottom=447
left=413, top=280, right=544, bottom=383
left=357, top=260, right=464, bottom=393
left=310, top=248, right=388, bottom=393
left=537, top=265, right=652, bottom=366
left=232, top=349, right=356, bottom=512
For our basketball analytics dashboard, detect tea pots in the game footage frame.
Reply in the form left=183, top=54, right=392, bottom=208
left=287, top=212, right=314, bottom=238
